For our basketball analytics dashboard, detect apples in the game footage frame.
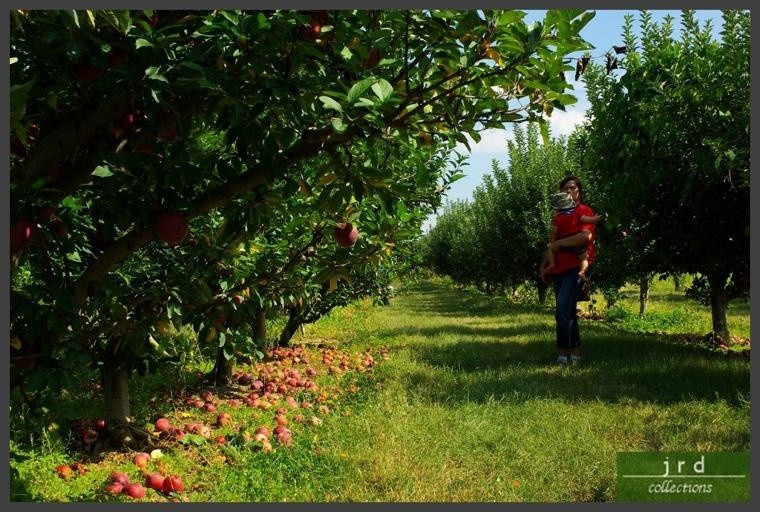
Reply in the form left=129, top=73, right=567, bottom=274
left=152, top=211, right=190, bottom=244
left=10, top=206, right=65, bottom=251
left=210, top=310, right=225, bottom=327
left=299, top=11, right=332, bottom=40
left=73, top=46, right=176, bottom=156
left=11, top=118, right=38, bottom=156
left=359, top=48, right=380, bottom=68
left=232, top=294, right=246, bottom=305
left=332, top=220, right=359, bottom=249
left=55, top=336, right=399, bottom=499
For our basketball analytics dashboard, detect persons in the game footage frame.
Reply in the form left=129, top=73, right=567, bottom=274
left=540, top=177, right=596, bottom=366
left=545, top=192, right=602, bottom=282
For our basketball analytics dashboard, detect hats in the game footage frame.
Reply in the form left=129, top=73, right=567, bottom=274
left=550, top=193, right=576, bottom=210
